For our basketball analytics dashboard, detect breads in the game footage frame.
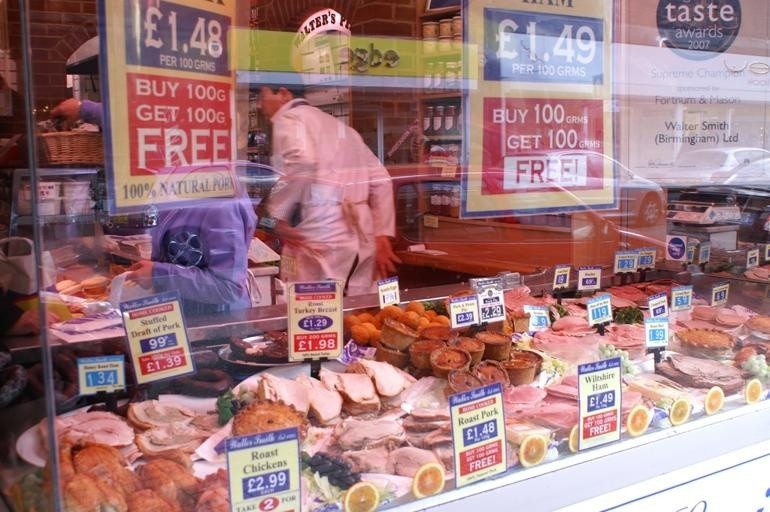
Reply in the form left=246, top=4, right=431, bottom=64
left=374, top=318, right=543, bottom=399
left=4, top=400, right=310, bottom=511
left=676, top=315, right=770, bottom=370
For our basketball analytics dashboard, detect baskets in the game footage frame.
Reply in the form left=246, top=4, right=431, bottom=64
left=38, top=132, right=105, bottom=166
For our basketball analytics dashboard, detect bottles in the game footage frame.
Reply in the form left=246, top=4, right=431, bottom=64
left=424, top=59, right=463, bottom=219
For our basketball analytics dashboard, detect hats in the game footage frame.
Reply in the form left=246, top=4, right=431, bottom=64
left=249, top=67, right=304, bottom=90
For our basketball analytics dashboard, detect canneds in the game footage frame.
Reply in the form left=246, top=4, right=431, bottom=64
left=423, top=16, right=463, bottom=218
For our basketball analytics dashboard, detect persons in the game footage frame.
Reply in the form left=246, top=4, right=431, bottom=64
left=51, top=98, right=259, bottom=400
left=248, top=61, right=404, bottom=304
left=409, top=121, right=429, bottom=162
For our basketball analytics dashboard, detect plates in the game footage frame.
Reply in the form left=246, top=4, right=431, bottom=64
left=744, top=267, right=770, bottom=281
left=15, top=334, right=417, bottom=498
